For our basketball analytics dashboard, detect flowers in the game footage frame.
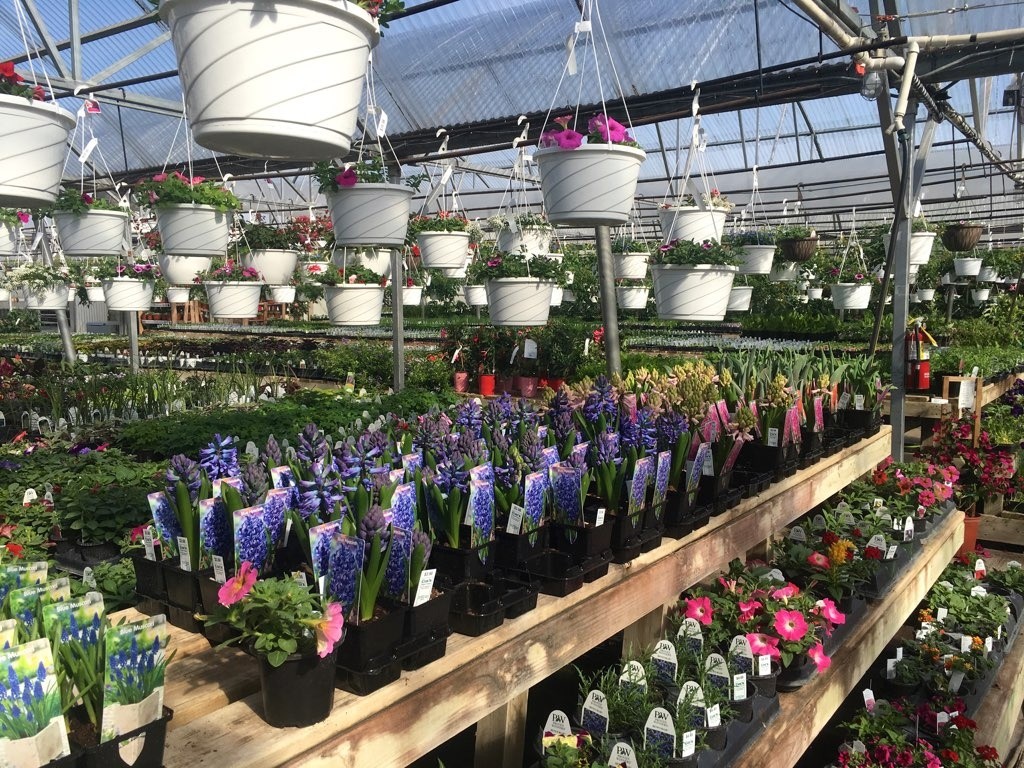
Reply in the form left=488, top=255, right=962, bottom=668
left=0, top=0, right=1024, bottom=307
left=0, top=327, right=1024, bottom=768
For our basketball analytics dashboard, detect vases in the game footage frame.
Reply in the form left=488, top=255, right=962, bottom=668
left=1005, top=275, right=1021, bottom=284
left=956, top=514, right=980, bottom=557
left=0, top=0, right=936, bottom=768
left=952, top=258, right=982, bottom=276
left=939, top=272, right=956, bottom=285
left=976, top=265, right=1000, bottom=281
left=995, top=276, right=1006, bottom=283
left=970, top=288, right=990, bottom=301
left=944, top=225, right=985, bottom=250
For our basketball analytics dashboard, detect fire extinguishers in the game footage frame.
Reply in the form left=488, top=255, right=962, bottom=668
left=907, top=316, right=930, bottom=389
